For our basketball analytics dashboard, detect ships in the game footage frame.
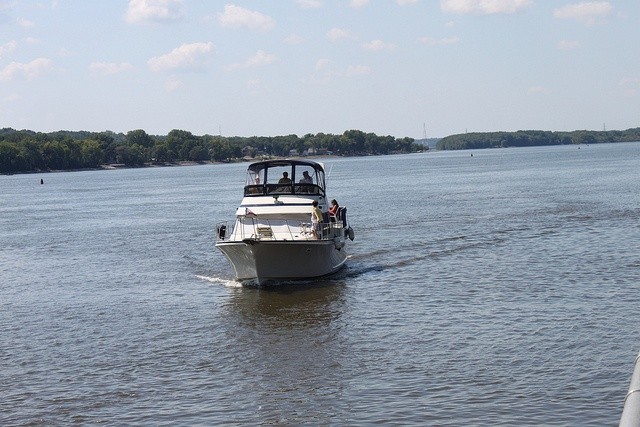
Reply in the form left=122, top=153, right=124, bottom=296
left=214, top=157, right=355, bottom=282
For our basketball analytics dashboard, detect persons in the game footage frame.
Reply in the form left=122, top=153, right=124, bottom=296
left=255, top=175, right=261, bottom=184
left=279, top=171, right=291, bottom=183
left=311, top=200, right=323, bottom=239
left=329, top=199, right=339, bottom=215
left=299, top=170, right=313, bottom=182
